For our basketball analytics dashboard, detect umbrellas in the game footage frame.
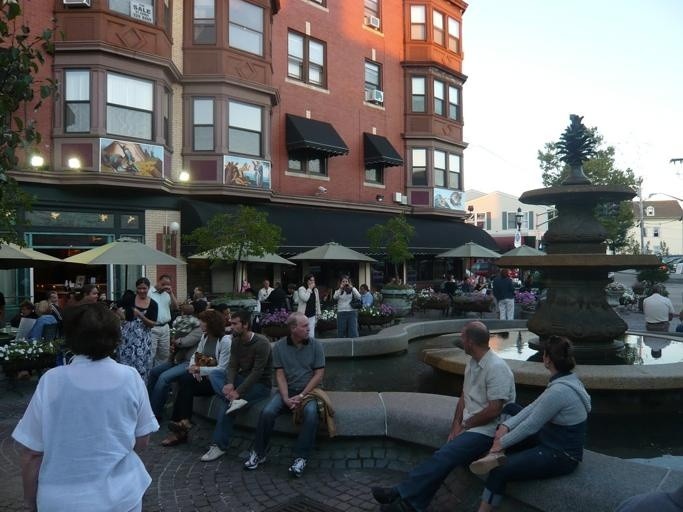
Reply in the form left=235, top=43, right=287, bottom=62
left=435, top=239, right=501, bottom=277
left=188, top=239, right=298, bottom=292
left=0, top=237, right=62, bottom=268
left=58, top=237, right=188, bottom=291
left=500, top=244, right=548, bottom=257
left=287, top=241, right=379, bottom=263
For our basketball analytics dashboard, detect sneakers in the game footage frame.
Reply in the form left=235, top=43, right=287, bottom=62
left=469, top=453, right=508, bottom=477
left=200, top=445, right=226, bottom=462
left=288, top=455, right=309, bottom=477
left=244, top=451, right=267, bottom=470
left=167, top=421, right=194, bottom=434
left=226, top=398, right=249, bottom=416
left=162, top=435, right=189, bottom=447
left=371, top=485, right=398, bottom=505
left=380, top=498, right=416, bottom=511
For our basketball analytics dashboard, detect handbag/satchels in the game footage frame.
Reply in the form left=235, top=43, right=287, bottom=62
left=350, top=294, right=363, bottom=309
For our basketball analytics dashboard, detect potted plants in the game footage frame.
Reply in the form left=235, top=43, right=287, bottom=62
left=366, top=211, right=416, bottom=320
left=632, top=261, right=669, bottom=314
left=175, top=203, right=288, bottom=327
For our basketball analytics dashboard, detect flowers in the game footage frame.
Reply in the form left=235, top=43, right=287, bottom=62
left=1, top=283, right=496, bottom=362
left=606, top=278, right=670, bottom=310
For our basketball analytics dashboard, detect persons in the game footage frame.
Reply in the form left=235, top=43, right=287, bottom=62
left=614, top=484, right=683, bottom=512
left=643, top=285, right=673, bottom=332
left=468, top=336, right=592, bottom=512
left=675, top=309, right=683, bottom=333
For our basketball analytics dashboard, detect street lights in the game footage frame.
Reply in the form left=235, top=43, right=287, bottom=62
left=515, top=206, right=524, bottom=232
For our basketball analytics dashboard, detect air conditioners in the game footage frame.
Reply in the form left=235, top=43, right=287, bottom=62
left=62, top=0, right=90, bottom=9
left=366, top=15, right=379, bottom=28
left=366, top=89, right=383, bottom=103
